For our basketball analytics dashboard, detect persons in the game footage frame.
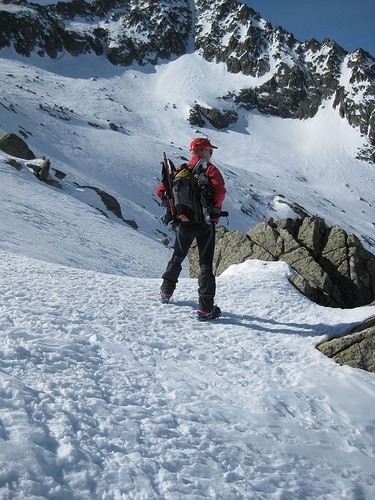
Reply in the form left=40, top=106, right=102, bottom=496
left=157, top=137, right=226, bottom=321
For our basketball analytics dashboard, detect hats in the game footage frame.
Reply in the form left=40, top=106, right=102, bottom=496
left=190, top=140, right=219, bottom=150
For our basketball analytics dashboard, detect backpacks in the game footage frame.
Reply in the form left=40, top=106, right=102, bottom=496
left=162, top=153, right=228, bottom=230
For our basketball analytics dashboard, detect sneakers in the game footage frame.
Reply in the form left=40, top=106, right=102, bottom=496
left=159, top=288, right=173, bottom=304
left=196, top=305, right=221, bottom=320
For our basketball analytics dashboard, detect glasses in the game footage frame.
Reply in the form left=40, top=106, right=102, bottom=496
left=203, top=147, right=214, bottom=155
